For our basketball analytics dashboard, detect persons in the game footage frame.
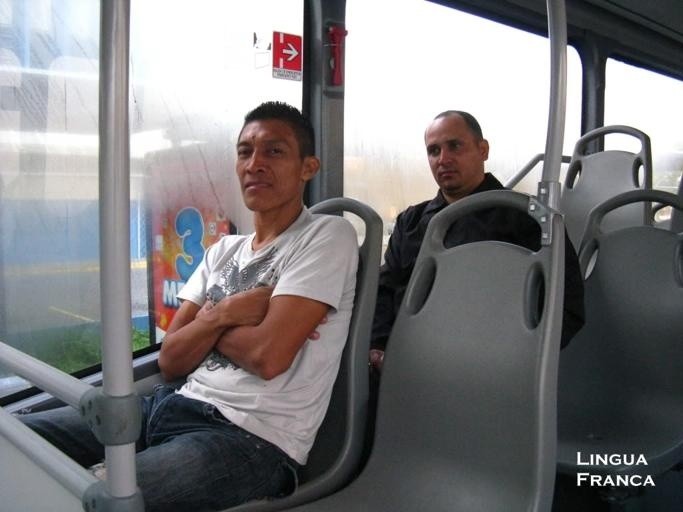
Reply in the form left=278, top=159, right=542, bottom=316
left=359, top=111, right=585, bottom=471
left=10, top=101, right=360, bottom=511
